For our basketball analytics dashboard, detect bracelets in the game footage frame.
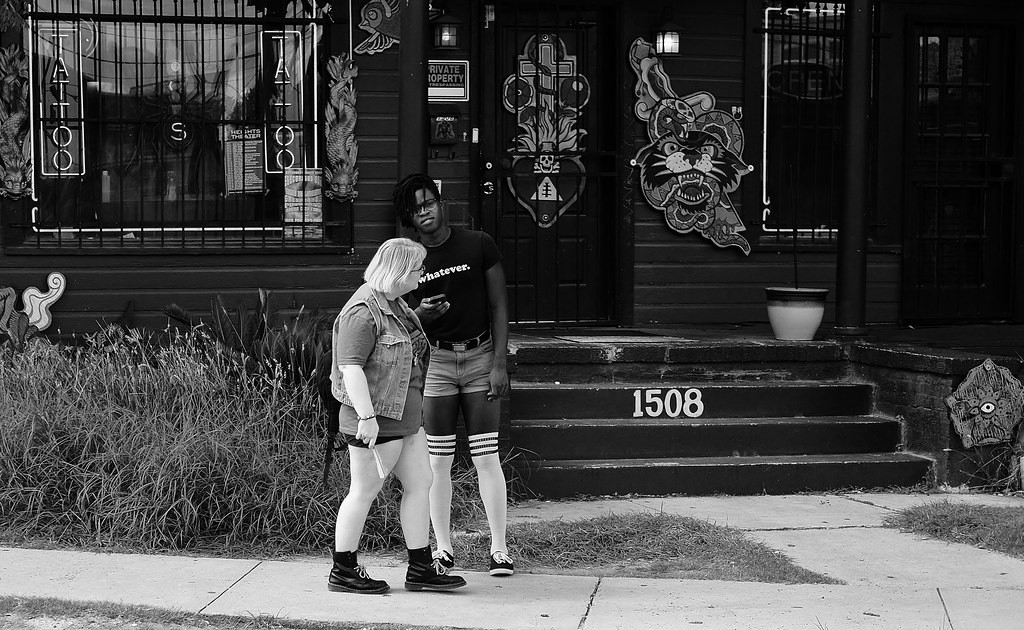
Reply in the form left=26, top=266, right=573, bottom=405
left=356, top=414, right=375, bottom=421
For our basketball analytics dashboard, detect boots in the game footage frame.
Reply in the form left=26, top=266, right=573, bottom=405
left=328, top=549, right=390, bottom=594
left=405, top=544, right=467, bottom=591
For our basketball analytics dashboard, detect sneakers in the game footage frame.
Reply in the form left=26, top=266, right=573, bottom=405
left=431, top=549, right=454, bottom=576
left=489, top=548, right=514, bottom=576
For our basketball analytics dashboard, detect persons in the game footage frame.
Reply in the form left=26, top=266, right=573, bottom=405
left=396, top=179, right=514, bottom=576
left=331, top=238, right=466, bottom=595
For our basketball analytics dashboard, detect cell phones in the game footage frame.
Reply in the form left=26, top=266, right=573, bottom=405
left=430, top=294, right=446, bottom=305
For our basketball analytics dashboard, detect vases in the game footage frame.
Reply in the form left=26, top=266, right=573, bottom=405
left=766, top=286, right=829, bottom=342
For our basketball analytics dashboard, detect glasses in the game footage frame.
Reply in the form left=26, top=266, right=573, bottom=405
left=411, top=265, right=426, bottom=275
left=407, top=197, right=438, bottom=217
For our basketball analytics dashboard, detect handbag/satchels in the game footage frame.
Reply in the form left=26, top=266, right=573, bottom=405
left=317, top=348, right=341, bottom=414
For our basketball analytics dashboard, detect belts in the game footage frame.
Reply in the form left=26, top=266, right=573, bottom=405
left=429, top=330, right=490, bottom=351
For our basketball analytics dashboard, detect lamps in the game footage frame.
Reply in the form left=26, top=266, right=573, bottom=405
left=431, top=14, right=470, bottom=55
left=652, top=21, right=686, bottom=57
